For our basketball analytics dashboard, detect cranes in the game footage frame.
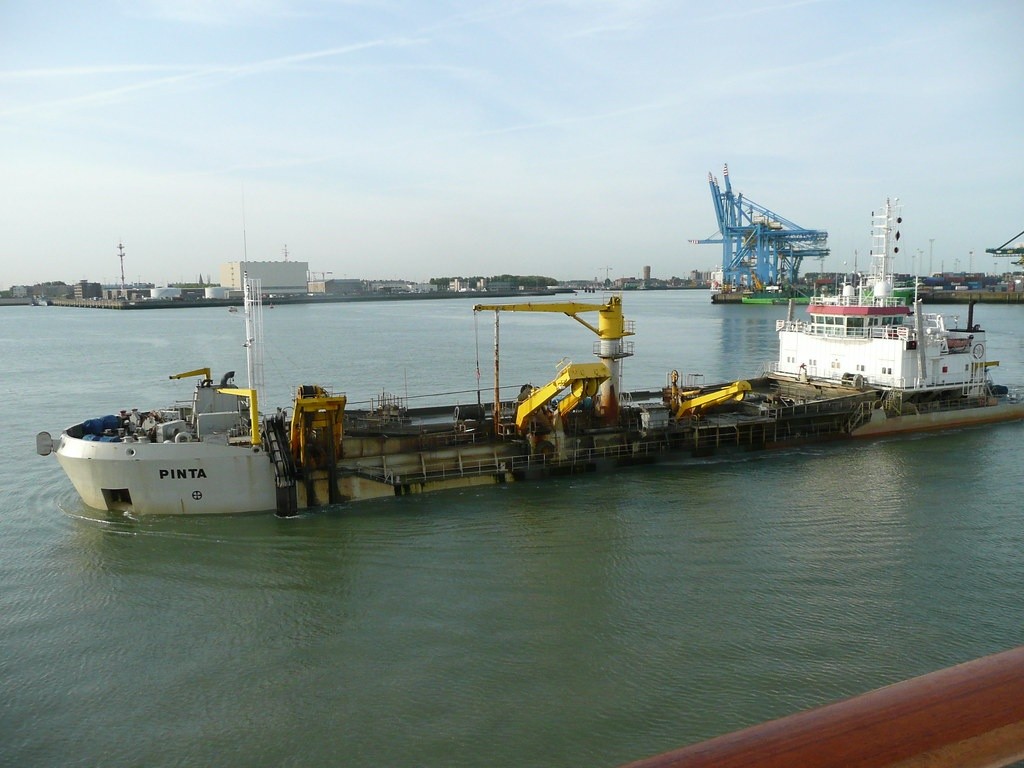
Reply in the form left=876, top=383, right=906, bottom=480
left=985, top=230, right=1024, bottom=265
left=686, top=164, right=831, bottom=292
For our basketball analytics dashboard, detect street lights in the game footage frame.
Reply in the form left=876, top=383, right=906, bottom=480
left=927, top=238, right=936, bottom=274
left=918, top=250, right=924, bottom=274
left=912, top=255, right=915, bottom=275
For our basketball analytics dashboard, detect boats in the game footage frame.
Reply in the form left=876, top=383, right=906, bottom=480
left=38, top=193, right=1024, bottom=517
